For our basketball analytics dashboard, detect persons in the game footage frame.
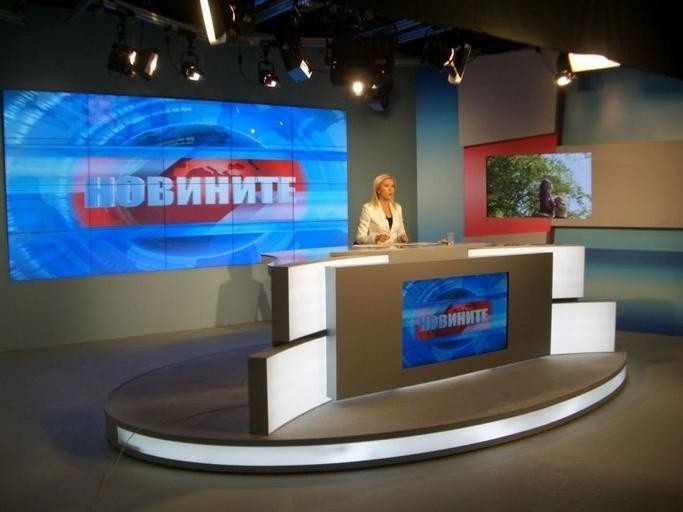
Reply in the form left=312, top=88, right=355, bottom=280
left=355, top=173, right=409, bottom=245
left=554, top=196, right=568, bottom=218
left=537, top=178, right=556, bottom=218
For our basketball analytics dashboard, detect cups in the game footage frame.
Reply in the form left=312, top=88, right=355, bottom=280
left=447, top=233, right=454, bottom=248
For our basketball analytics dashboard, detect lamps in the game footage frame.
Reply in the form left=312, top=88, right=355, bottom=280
left=349, top=44, right=395, bottom=105
left=323, top=27, right=359, bottom=90
left=275, top=26, right=316, bottom=87
left=195, top=1, right=247, bottom=50
left=178, top=61, right=204, bottom=85
left=553, top=46, right=620, bottom=89
left=112, top=37, right=161, bottom=83
left=421, top=35, right=473, bottom=88
left=254, top=59, right=278, bottom=90
